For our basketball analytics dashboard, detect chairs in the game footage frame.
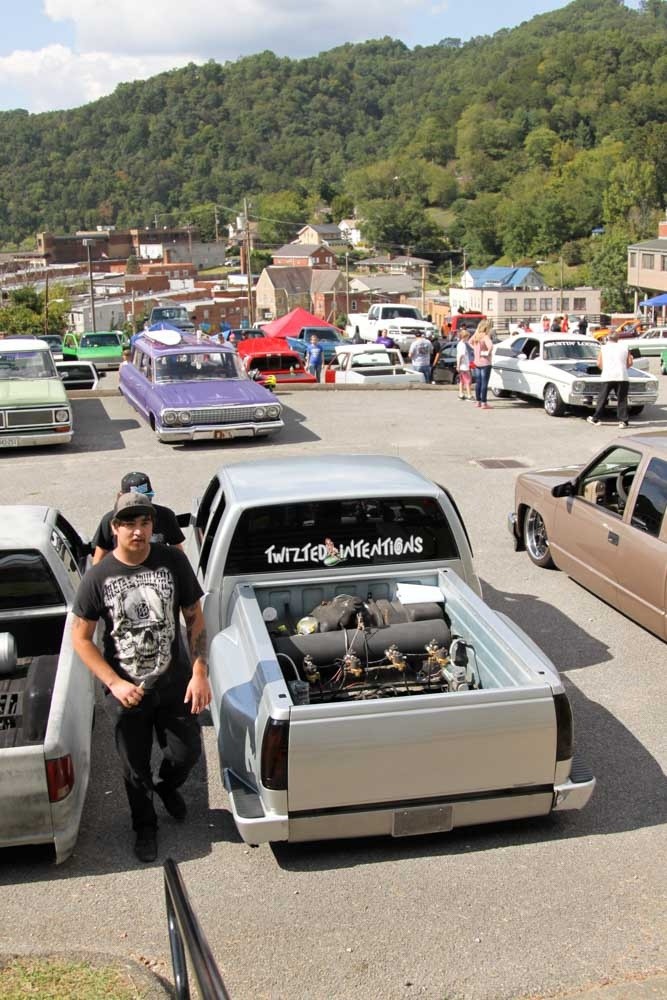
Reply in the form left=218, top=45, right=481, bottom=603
left=529, top=346, right=539, bottom=359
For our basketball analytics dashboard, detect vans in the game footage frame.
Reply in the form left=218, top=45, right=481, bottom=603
left=447, top=313, right=489, bottom=342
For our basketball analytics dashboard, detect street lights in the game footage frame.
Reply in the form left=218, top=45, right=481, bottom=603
left=82, top=238, right=96, bottom=334
left=535, top=256, right=564, bottom=313
left=345, top=252, right=349, bottom=314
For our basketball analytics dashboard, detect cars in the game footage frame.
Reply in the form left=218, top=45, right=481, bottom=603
left=117, top=333, right=285, bottom=444
left=223, top=320, right=271, bottom=343
left=1, top=339, right=74, bottom=448
left=143, top=305, right=195, bottom=335
left=508, top=312, right=667, bottom=376
left=62, top=330, right=130, bottom=370
left=487, top=331, right=658, bottom=418
left=507, top=429, right=667, bottom=642
left=37, top=335, right=64, bottom=360
left=432, top=340, right=477, bottom=384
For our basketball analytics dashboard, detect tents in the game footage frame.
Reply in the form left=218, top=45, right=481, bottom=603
left=639, top=294, right=667, bottom=326
left=130, top=320, right=193, bottom=361
left=259, top=307, right=343, bottom=338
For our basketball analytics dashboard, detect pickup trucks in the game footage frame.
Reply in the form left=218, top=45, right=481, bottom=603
left=286, top=327, right=352, bottom=363
left=54, top=360, right=119, bottom=391
left=319, top=344, right=427, bottom=385
left=345, top=303, right=437, bottom=353
left=174, top=454, right=596, bottom=847
left=237, top=338, right=318, bottom=385
left=0, top=504, right=98, bottom=865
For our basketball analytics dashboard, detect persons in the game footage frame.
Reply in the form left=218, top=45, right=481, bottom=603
left=306, top=335, right=324, bottom=383
left=214, top=330, right=247, bottom=348
left=376, top=330, right=393, bottom=347
left=71, top=492, right=212, bottom=862
left=587, top=331, right=633, bottom=428
left=456, top=320, right=493, bottom=409
left=408, top=331, right=440, bottom=384
left=91, top=472, right=185, bottom=565
left=518, top=313, right=588, bottom=335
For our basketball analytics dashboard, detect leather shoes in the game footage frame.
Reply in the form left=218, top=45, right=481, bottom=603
left=150, top=771, right=187, bottom=819
left=134, top=832, right=158, bottom=862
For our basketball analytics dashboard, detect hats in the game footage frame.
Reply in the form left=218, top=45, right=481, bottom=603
left=460, top=323, right=465, bottom=326
left=426, top=330, right=432, bottom=338
left=415, top=331, right=422, bottom=337
left=120, top=471, right=156, bottom=497
left=113, top=493, right=157, bottom=519
left=120, top=472, right=154, bottom=496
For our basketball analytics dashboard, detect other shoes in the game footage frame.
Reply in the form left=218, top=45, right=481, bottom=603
left=458, top=395, right=467, bottom=400
left=467, top=395, right=476, bottom=401
left=481, top=404, right=494, bottom=409
left=586, top=416, right=601, bottom=425
left=475, top=402, right=481, bottom=406
left=619, top=421, right=627, bottom=428
